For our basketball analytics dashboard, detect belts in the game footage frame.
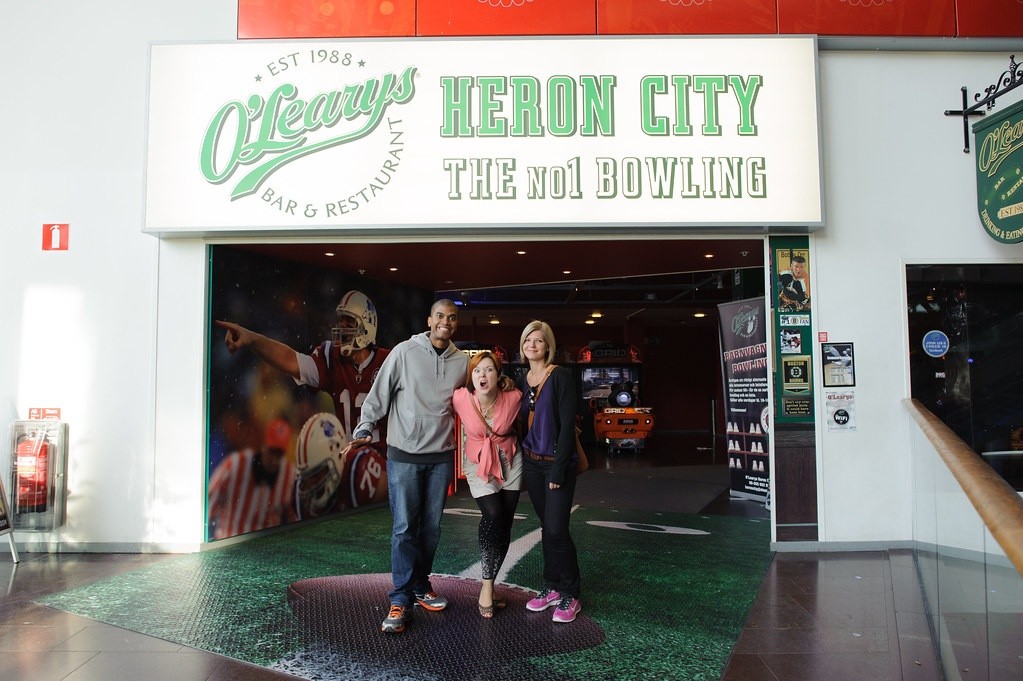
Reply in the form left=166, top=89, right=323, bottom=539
left=524, top=448, right=555, bottom=461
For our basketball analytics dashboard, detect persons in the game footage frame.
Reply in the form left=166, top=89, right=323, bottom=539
left=295, top=412, right=388, bottom=518
left=208, top=419, right=297, bottom=540
left=215, top=291, right=391, bottom=459
left=779, top=256, right=810, bottom=305
left=453, top=352, right=524, bottom=618
left=340, top=298, right=471, bottom=631
left=609, top=381, right=636, bottom=407
left=516, top=321, right=581, bottom=622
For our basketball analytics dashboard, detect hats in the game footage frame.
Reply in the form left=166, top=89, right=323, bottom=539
left=263, top=418, right=293, bottom=451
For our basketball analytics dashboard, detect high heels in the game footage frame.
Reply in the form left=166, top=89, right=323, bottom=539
left=493, top=595, right=506, bottom=607
left=478, top=595, right=494, bottom=618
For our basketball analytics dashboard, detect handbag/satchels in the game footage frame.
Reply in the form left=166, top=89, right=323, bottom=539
left=574, top=426, right=588, bottom=474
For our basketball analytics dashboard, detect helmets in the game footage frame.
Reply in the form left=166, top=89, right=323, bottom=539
left=334, top=290, right=378, bottom=356
left=294, top=413, right=348, bottom=517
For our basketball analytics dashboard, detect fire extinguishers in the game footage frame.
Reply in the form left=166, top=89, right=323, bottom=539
left=17, top=431, right=50, bottom=512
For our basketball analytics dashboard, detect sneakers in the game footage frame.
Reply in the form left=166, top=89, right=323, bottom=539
left=526, top=586, right=563, bottom=611
left=414, top=590, right=447, bottom=610
left=552, top=595, right=581, bottom=622
left=381, top=605, right=407, bottom=633
